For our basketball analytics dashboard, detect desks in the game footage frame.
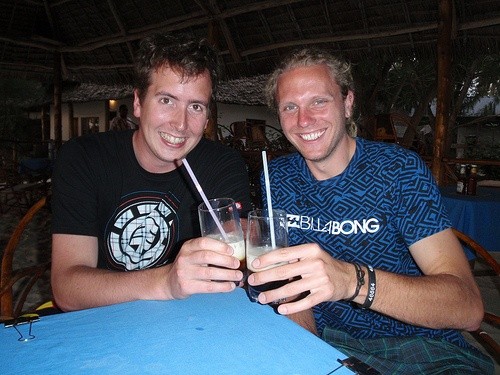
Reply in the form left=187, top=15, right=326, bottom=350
left=0, top=286, right=378, bottom=375
left=440, top=185, right=500, bottom=262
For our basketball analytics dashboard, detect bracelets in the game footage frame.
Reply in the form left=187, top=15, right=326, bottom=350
left=345, top=262, right=377, bottom=311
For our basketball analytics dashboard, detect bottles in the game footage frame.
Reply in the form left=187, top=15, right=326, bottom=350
left=467, top=165, right=477, bottom=193
left=457, top=165, right=466, bottom=194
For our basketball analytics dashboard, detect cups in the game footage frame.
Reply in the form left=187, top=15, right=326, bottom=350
left=246, top=209, right=289, bottom=299
left=198, top=198, right=247, bottom=282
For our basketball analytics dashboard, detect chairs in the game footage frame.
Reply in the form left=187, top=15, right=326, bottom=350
left=0, top=191, right=61, bottom=324
left=450, top=226, right=500, bottom=368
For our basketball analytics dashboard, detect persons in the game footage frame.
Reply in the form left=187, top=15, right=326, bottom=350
left=260, top=46, right=500, bottom=375
left=49, top=30, right=261, bottom=315
left=109, top=104, right=132, bottom=132
